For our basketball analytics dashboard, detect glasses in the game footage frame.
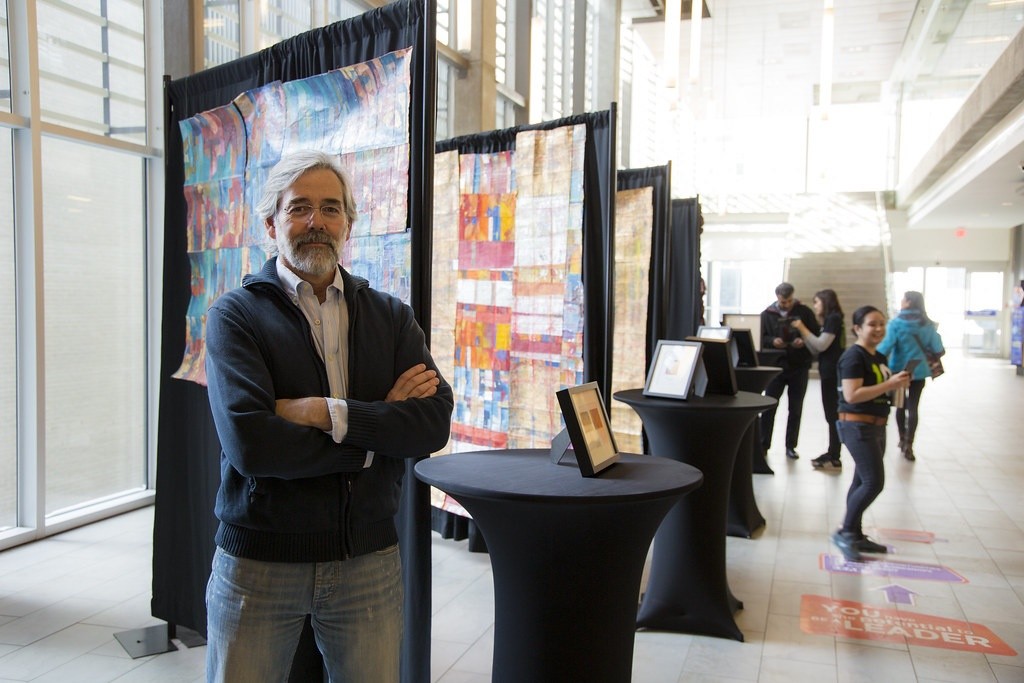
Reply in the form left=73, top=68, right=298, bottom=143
left=273, top=203, right=350, bottom=217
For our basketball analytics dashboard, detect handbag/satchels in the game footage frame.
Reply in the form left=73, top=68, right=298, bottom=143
left=925, top=350, right=943, bottom=380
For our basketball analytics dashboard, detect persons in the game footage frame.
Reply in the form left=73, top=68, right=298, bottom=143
left=789, top=288, right=848, bottom=468
left=830, top=306, right=913, bottom=563
left=760, top=282, right=821, bottom=459
left=876, top=289, right=945, bottom=461
left=203, top=150, right=458, bottom=683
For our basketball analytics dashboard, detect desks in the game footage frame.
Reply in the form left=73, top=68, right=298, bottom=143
left=414, top=449, right=701, bottom=683
left=758, top=349, right=788, bottom=366
left=732, top=366, right=783, bottom=475
left=613, top=389, right=777, bottom=643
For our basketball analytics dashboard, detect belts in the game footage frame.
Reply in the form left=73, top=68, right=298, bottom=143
left=839, top=412, right=888, bottom=426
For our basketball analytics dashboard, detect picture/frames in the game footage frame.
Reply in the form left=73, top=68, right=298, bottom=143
left=723, top=313, right=763, bottom=352
left=642, top=340, right=702, bottom=399
left=556, top=382, right=620, bottom=477
left=696, top=325, right=733, bottom=340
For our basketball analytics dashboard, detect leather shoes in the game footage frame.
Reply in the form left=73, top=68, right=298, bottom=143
left=833, top=531, right=864, bottom=563
left=837, top=529, right=887, bottom=553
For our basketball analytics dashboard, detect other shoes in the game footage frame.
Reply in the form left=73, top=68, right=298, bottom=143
left=811, top=452, right=842, bottom=470
left=906, top=449, right=915, bottom=461
left=787, top=449, right=799, bottom=458
left=899, top=441, right=905, bottom=452
